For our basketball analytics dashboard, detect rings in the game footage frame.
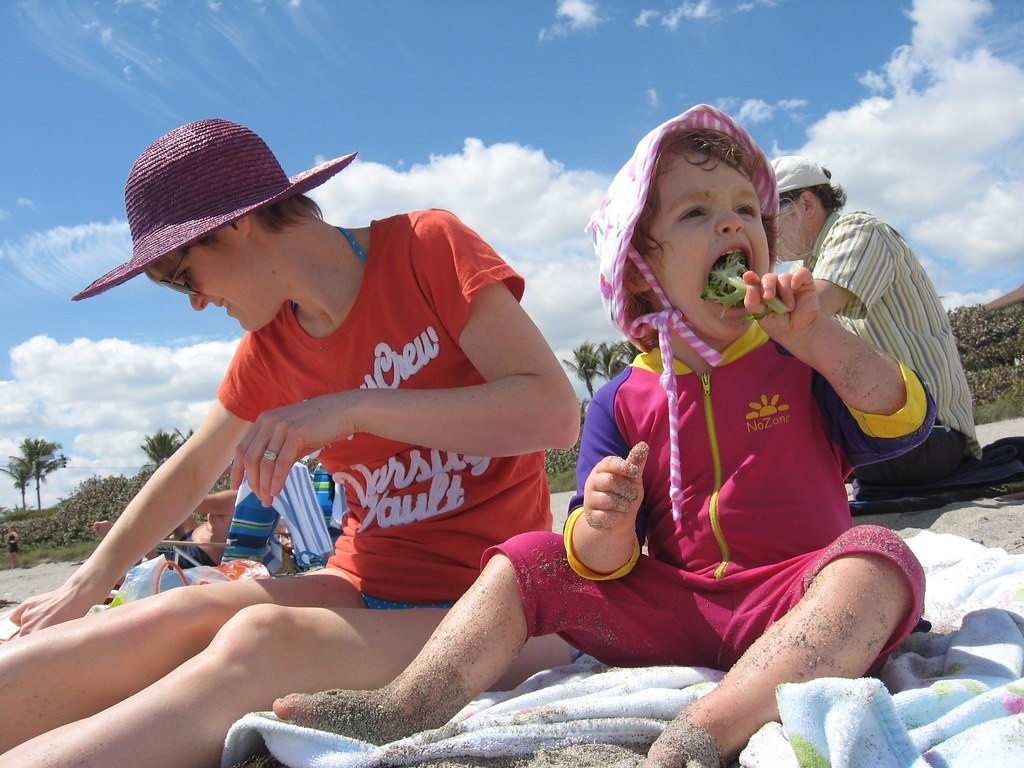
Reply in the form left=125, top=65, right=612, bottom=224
left=264, top=450, right=276, bottom=460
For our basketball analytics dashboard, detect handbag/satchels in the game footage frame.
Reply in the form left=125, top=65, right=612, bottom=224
left=106, top=553, right=272, bottom=610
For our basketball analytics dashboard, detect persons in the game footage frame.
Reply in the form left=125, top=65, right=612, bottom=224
left=767, top=154, right=982, bottom=489
left=94, top=457, right=239, bottom=570
left=272, top=105, right=938, bottom=768
left=7, top=526, right=21, bottom=568
left=0, top=121, right=582, bottom=768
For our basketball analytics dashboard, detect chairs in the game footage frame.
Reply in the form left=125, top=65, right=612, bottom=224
left=104, top=459, right=348, bottom=604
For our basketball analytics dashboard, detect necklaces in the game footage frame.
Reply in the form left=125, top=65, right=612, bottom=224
left=290, top=226, right=367, bottom=311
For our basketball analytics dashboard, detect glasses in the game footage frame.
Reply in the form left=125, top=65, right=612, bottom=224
left=159, top=245, right=201, bottom=296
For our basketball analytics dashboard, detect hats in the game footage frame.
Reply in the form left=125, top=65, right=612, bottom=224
left=770, top=155, right=833, bottom=195
left=69, top=118, right=359, bottom=302
left=584, top=104, right=779, bottom=355
